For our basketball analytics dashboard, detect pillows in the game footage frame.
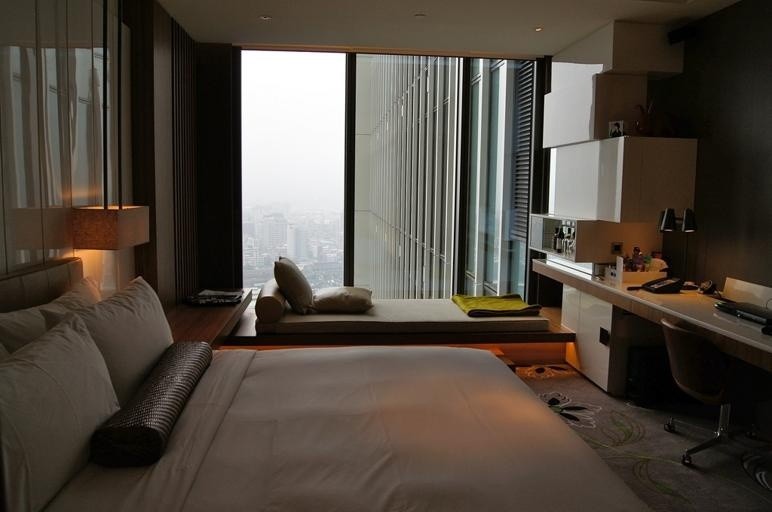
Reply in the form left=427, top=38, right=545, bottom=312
left=274, top=257, right=314, bottom=316
left=312, top=287, right=374, bottom=314
left=3, top=275, right=103, bottom=357
left=255, top=277, right=288, bottom=322
left=2, top=310, right=120, bottom=509
left=97, top=340, right=213, bottom=471
left=38, top=274, right=176, bottom=409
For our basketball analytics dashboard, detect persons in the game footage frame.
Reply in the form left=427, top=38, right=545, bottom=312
left=610, top=122, right=621, bottom=137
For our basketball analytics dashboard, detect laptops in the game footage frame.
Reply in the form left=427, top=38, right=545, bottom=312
left=714, top=301, right=772, bottom=326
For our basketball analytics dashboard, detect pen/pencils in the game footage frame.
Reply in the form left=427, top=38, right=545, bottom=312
left=713, top=303, right=767, bottom=325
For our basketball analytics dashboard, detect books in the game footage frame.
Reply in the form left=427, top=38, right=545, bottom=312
left=191, top=289, right=244, bottom=302
left=734, top=302, right=772, bottom=326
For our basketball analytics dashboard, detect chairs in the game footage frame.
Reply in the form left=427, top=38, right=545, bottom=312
left=659, top=318, right=770, bottom=466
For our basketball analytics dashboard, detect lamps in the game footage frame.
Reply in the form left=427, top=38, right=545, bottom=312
left=657, top=206, right=700, bottom=283
left=72, top=1, right=153, bottom=253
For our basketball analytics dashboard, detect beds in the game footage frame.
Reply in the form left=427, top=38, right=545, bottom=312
left=3, top=257, right=650, bottom=508
left=255, top=259, right=551, bottom=341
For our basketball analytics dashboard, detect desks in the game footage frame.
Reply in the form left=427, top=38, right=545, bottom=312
left=167, top=288, right=253, bottom=350
left=531, top=256, right=771, bottom=377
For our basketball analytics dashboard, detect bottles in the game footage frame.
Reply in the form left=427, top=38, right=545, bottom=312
left=552, top=227, right=576, bottom=256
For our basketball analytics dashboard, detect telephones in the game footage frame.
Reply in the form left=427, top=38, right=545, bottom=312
left=642, top=275, right=685, bottom=293
